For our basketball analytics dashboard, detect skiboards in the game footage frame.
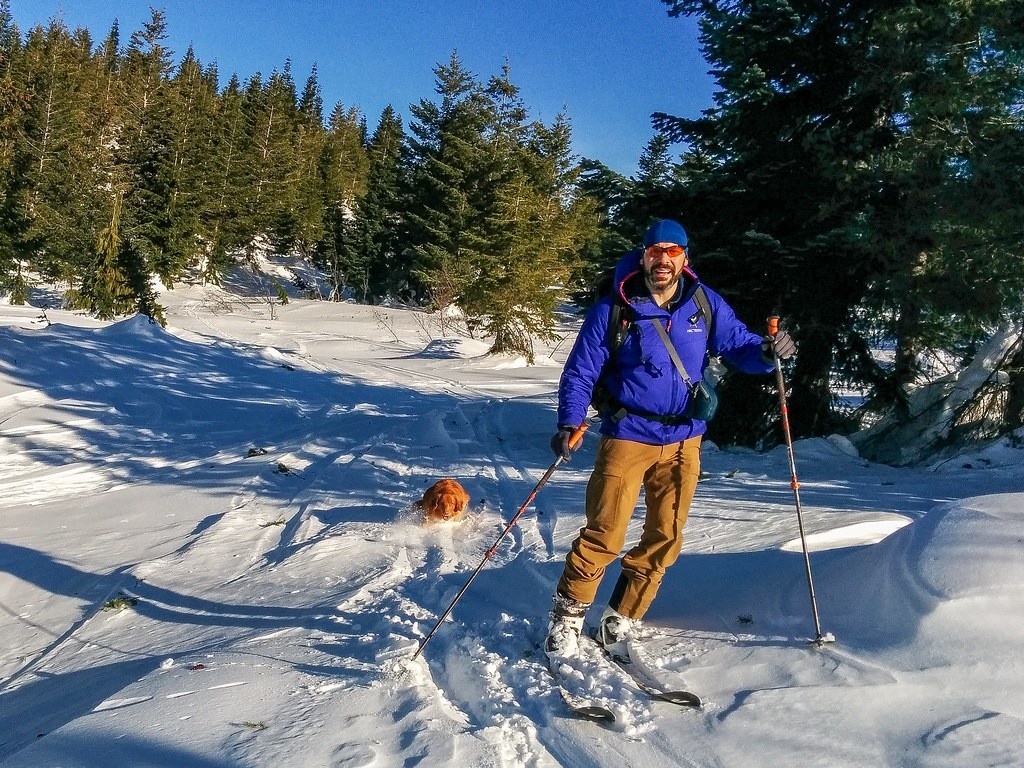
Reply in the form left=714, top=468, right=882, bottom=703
left=544, top=624, right=702, bottom=720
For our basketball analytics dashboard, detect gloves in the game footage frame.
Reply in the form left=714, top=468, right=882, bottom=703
left=761, top=331, right=796, bottom=362
left=550, top=427, right=583, bottom=465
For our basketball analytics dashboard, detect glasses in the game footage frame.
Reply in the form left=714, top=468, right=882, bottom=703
left=644, top=244, right=685, bottom=257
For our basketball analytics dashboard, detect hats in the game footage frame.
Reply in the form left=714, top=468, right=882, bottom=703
left=642, top=219, right=688, bottom=248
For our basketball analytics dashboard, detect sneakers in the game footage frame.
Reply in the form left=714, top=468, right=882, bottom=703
left=543, top=590, right=592, bottom=667
left=594, top=603, right=643, bottom=664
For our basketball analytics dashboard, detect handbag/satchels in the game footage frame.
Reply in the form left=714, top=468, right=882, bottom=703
left=689, top=381, right=718, bottom=421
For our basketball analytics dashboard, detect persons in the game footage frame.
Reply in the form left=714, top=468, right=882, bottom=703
left=546, top=219, right=796, bottom=664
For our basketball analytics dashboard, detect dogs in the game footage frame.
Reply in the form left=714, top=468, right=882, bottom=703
left=412, top=477, right=471, bottom=528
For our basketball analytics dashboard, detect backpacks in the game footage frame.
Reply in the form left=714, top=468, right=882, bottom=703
left=590, top=278, right=713, bottom=421
